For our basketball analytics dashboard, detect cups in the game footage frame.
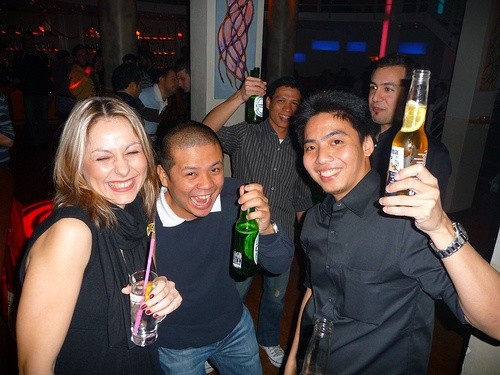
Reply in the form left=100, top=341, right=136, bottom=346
left=129, top=270, right=159, bottom=347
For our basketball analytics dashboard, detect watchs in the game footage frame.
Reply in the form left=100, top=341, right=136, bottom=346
left=272, top=222, right=278, bottom=234
left=430, top=221, right=469, bottom=258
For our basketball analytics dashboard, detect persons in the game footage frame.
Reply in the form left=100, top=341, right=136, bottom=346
left=16, top=96, right=183, bottom=375
left=14, top=31, right=191, bottom=165
left=369, top=55, right=452, bottom=208
left=202, top=76, right=313, bottom=373
left=0, top=91, right=15, bottom=168
left=155, top=120, right=294, bottom=375
left=284, top=90, right=500, bottom=375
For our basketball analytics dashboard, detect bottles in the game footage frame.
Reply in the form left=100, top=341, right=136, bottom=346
left=384, top=69, right=430, bottom=196
left=300, top=318, right=333, bottom=375
left=229, top=183, right=259, bottom=281
left=245, top=67, right=263, bottom=123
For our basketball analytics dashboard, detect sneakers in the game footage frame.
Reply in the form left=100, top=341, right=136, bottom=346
left=205, top=361, right=214, bottom=374
left=257, top=341, right=285, bottom=367
